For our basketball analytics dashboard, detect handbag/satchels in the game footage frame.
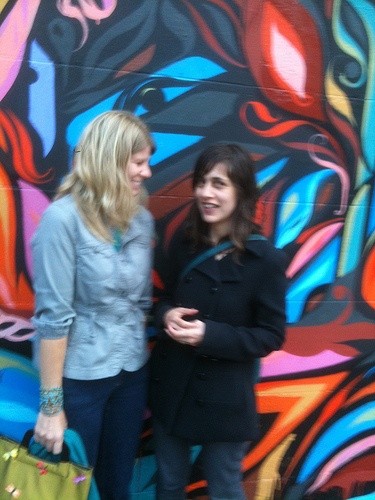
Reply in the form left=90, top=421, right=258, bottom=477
left=0, top=430, right=94, bottom=500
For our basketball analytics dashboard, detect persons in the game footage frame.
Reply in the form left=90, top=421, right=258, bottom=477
left=152, top=143, right=288, bottom=500
left=0, top=110, right=155, bottom=500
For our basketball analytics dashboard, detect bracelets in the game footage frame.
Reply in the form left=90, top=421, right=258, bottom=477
left=40, top=387, right=64, bottom=416
